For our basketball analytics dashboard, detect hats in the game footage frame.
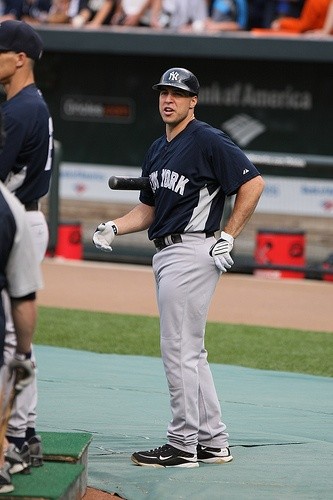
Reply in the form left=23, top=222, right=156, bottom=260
left=0, top=18, right=43, bottom=62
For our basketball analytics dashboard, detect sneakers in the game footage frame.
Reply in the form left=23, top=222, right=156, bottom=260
left=131, top=443, right=200, bottom=468
left=197, top=443, right=233, bottom=465
left=0, top=434, right=43, bottom=494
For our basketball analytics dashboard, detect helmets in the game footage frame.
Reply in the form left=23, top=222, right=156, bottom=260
left=151, top=67, right=200, bottom=97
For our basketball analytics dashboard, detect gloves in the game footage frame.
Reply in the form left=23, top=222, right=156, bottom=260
left=6, top=351, right=37, bottom=393
left=91, top=220, right=118, bottom=253
left=209, top=230, right=235, bottom=273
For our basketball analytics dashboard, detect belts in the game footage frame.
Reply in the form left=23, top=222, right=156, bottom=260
left=22, top=202, right=38, bottom=212
left=153, top=234, right=183, bottom=251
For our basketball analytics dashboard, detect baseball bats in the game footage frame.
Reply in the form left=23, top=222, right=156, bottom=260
left=109, top=174, right=149, bottom=191
left=0, top=366, right=27, bottom=457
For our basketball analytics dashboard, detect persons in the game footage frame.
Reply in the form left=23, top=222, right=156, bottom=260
left=92, top=67, right=265, bottom=468
left=0, top=0, right=333, bottom=35
left=0, top=20, right=55, bottom=475
left=0, top=178, right=42, bottom=493
